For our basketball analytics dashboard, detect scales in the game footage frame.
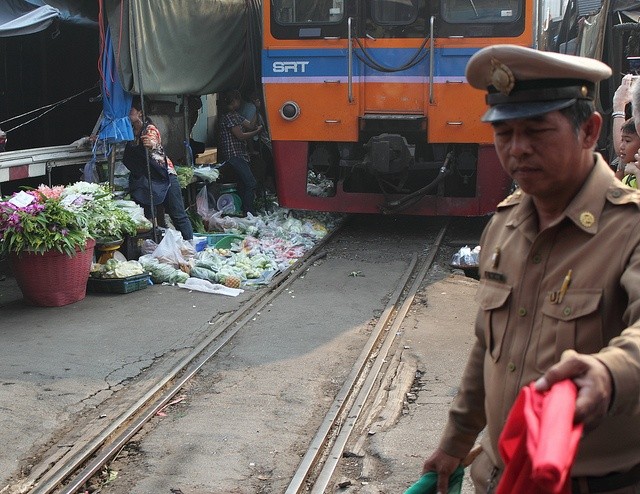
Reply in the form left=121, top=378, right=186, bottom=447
left=217, top=183, right=243, bottom=217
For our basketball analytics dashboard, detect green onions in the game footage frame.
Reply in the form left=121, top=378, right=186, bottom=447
left=185, top=209, right=206, bottom=233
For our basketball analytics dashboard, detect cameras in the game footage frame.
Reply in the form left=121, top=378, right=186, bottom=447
left=630, top=75, right=640, bottom=87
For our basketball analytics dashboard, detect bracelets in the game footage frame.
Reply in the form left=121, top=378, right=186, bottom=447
left=614, top=115, right=626, bottom=118
left=611, top=112, right=627, bottom=116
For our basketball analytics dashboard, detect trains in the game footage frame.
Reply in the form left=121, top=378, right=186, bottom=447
left=261, top=1, right=543, bottom=218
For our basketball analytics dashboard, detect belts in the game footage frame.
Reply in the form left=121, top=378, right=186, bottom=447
left=570, top=463, right=639, bottom=492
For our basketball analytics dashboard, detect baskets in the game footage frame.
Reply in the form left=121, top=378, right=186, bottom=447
left=193, top=232, right=234, bottom=245
left=214, top=235, right=244, bottom=248
left=87, top=271, right=150, bottom=293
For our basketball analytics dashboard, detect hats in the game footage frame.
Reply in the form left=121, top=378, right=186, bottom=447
left=464, top=43, right=613, bottom=122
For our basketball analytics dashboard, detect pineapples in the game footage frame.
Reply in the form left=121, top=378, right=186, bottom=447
left=218, top=274, right=241, bottom=289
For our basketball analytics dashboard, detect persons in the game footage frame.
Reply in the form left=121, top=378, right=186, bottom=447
left=216, top=90, right=265, bottom=216
left=619, top=117, right=640, bottom=164
left=627, top=78, right=639, bottom=138
left=623, top=162, right=640, bottom=189
left=248, top=79, right=274, bottom=168
left=121, top=96, right=193, bottom=245
left=188, top=95, right=203, bottom=135
left=634, top=148, right=639, bottom=169
left=611, top=73, right=632, bottom=157
left=421, top=44, right=640, bottom=493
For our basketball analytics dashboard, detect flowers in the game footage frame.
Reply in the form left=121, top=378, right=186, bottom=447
left=0, top=183, right=92, bottom=259
left=92, top=192, right=138, bottom=242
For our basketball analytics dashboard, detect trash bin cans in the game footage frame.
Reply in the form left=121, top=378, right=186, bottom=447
left=123, top=232, right=140, bottom=261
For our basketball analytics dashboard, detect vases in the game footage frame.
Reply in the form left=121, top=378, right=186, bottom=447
left=96, top=239, right=124, bottom=264
left=11, top=238, right=95, bottom=307
